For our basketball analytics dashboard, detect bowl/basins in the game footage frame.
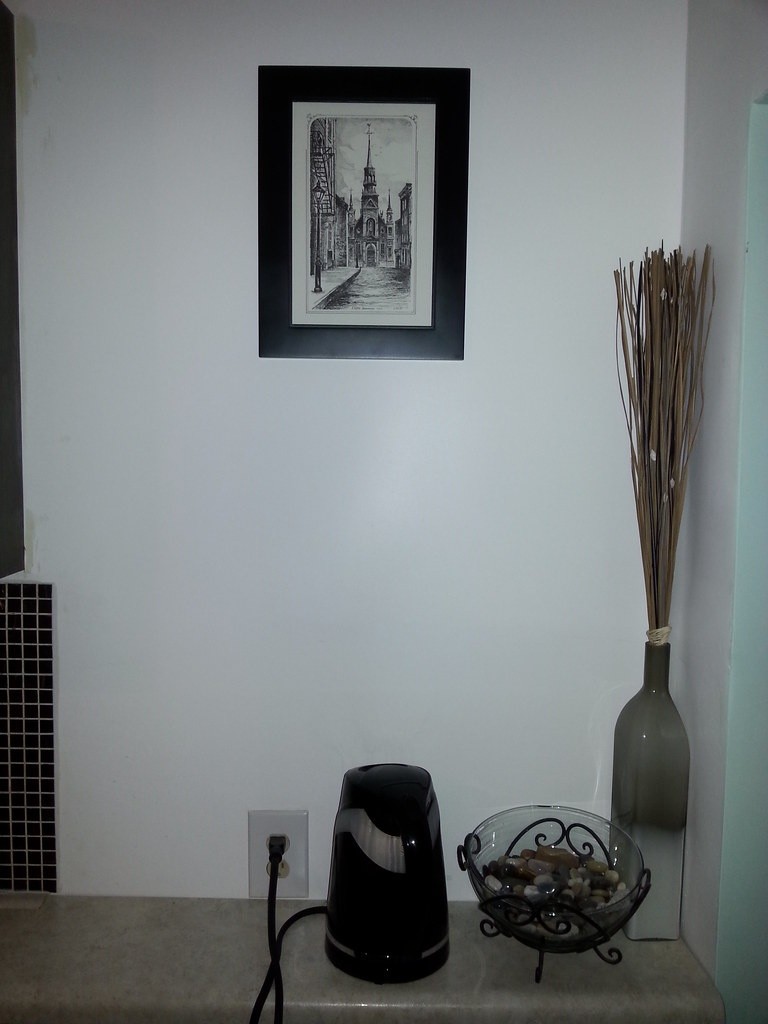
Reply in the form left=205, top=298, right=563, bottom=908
left=465, top=805, right=644, bottom=939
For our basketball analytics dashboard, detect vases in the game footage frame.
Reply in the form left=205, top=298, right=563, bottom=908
left=608, top=643, right=691, bottom=942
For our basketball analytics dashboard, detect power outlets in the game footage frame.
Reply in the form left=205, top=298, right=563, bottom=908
left=249, top=809, right=309, bottom=901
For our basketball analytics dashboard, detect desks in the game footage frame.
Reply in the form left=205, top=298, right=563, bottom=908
left=0, top=889, right=728, bottom=1024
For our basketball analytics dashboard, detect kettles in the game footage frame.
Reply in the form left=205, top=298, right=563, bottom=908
left=325, top=763, right=449, bottom=983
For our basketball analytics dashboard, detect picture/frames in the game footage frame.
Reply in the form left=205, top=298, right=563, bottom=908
left=257, top=65, right=472, bottom=360
